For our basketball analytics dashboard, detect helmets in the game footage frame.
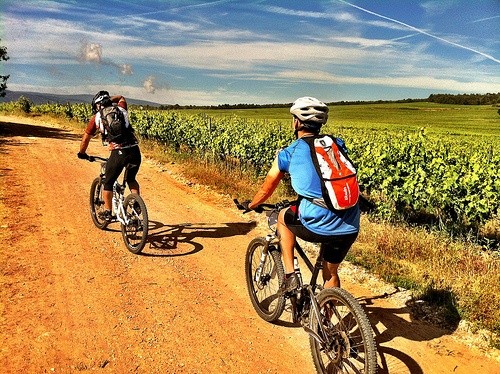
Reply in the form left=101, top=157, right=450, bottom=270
left=289, top=96, right=330, bottom=125
left=93, top=90, right=111, bottom=103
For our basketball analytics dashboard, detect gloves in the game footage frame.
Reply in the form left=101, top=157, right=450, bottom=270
left=77, top=152, right=88, bottom=159
left=240, top=201, right=254, bottom=214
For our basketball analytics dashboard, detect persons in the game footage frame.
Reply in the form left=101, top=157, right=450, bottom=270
left=77, top=90, right=141, bottom=227
left=242, top=97, right=360, bottom=324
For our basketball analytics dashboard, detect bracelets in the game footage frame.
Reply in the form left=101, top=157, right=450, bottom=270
left=249, top=203, right=253, bottom=210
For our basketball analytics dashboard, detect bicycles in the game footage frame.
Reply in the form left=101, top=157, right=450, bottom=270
left=233, top=197, right=378, bottom=374
left=80, top=154, right=149, bottom=254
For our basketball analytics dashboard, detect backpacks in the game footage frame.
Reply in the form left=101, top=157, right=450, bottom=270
left=100, top=106, right=128, bottom=142
left=300, top=134, right=359, bottom=211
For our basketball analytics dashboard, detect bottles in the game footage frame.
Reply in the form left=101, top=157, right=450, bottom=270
left=294, top=257, right=303, bottom=287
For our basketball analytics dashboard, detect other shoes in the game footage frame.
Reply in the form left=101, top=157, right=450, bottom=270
left=98, top=211, right=113, bottom=218
left=132, top=205, right=142, bottom=216
left=277, top=274, right=300, bottom=296
